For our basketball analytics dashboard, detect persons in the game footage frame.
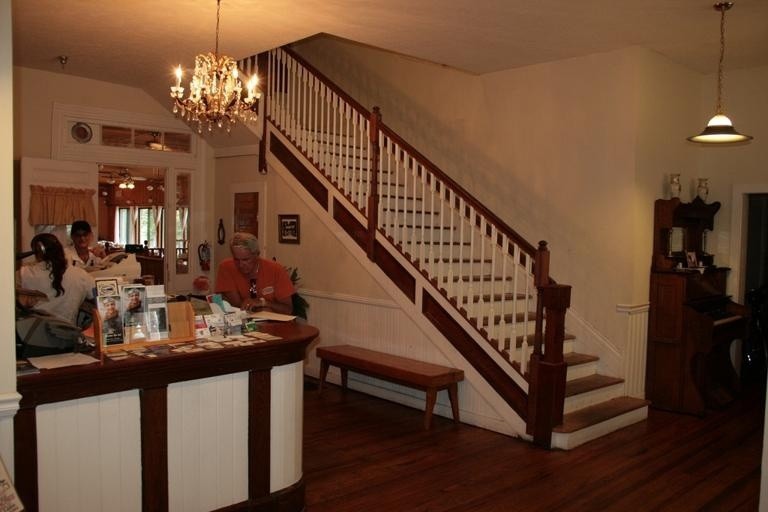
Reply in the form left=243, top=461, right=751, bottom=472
left=16, top=233, right=94, bottom=361
left=65, top=220, right=101, bottom=268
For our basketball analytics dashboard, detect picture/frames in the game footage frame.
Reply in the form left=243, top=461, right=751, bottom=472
left=278, top=214, right=300, bottom=244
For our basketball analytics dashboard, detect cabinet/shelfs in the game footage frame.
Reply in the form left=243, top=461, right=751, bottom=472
left=646, top=198, right=744, bottom=416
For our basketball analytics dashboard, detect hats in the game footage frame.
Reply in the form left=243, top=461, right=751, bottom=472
left=70, top=220, right=93, bottom=236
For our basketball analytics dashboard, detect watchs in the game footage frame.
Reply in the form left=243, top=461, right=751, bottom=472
left=259, top=297, right=266, bottom=307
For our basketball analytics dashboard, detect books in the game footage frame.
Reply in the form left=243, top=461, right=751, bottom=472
left=94, top=278, right=169, bottom=347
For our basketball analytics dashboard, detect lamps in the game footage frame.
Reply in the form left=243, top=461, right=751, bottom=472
left=685, top=3, right=755, bottom=145
left=169, top=1, right=262, bottom=134
left=119, top=169, right=135, bottom=189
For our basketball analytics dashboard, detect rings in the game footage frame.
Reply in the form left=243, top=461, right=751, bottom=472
left=246, top=304, right=249, bottom=307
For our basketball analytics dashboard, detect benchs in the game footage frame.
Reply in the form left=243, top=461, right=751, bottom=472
left=314, top=345, right=463, bottom=431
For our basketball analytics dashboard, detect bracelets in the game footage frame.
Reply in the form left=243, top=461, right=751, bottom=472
left=214, top=231, right=298, bottom=315
